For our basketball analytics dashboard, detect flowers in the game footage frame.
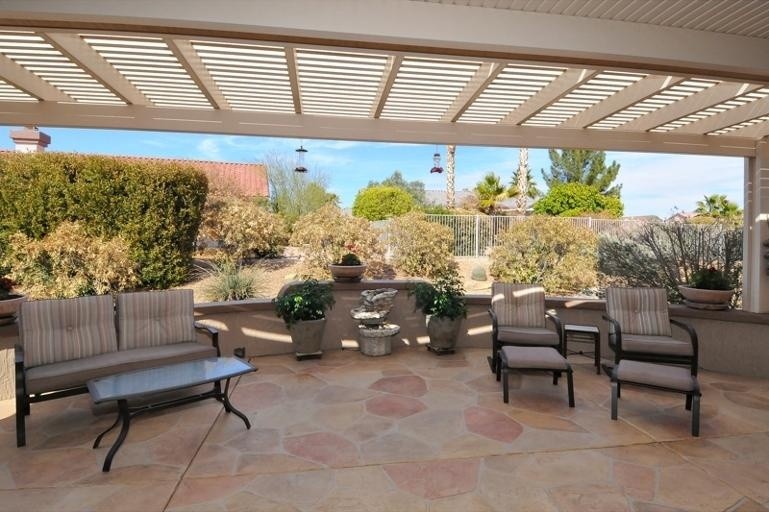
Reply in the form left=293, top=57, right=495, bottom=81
left=0, top=277, right=16, bottom=300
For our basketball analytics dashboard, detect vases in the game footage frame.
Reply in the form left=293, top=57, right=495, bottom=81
left=0, top=295, right=26, bottom=317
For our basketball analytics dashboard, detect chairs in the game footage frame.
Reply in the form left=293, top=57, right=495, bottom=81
left=487, top=282, right=564, bottom=378
left=601, top=286, right=698, bottom=410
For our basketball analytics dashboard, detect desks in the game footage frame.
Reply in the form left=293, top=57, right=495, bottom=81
left=562, top=324, right=601, bottom=375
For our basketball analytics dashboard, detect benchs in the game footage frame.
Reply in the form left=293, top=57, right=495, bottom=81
left=14, top=288, right=222, bottom=448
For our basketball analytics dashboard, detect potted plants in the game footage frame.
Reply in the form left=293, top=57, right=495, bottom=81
left=679, top=267, right=736, bottom=303
left=271, top=277, right=336, bottom=352
left=330, top=253, right=367, bottom=278
left=408, top=277, right=467, bottom=348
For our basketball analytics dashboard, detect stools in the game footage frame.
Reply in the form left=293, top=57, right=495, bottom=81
left=495, top=346, right=575, bottom=407
left=611, top=359, right=700, bottom=436
